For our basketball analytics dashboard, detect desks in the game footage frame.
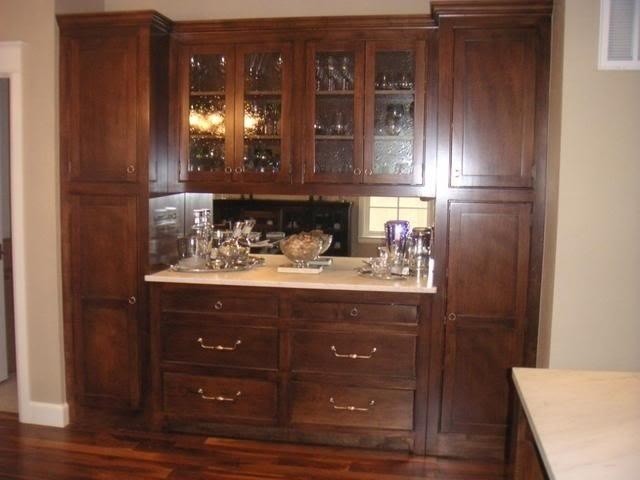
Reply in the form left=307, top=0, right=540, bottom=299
left=510, top=365, right=640, bottom=480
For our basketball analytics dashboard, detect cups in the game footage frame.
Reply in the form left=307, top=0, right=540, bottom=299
left=384, top=218, right=410, bottom=276
left=410, top=227, right=434, bottom=276
left=370, top=257, right=393, bottom=279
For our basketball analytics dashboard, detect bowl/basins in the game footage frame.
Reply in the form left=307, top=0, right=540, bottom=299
left=319, top=234, right=332, bottom=255
left=280, top=236, right=321, bottom=266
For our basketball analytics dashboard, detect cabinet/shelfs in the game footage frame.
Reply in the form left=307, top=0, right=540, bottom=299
left=54, top=10, right=184, bottom=431
left=212, top=201, right=355, bottom=256
left=147, top=254, right=433, bottom=461
left=429, top=0, right=555, bottom=462
left=176, top=14, right=439, bottom=197
